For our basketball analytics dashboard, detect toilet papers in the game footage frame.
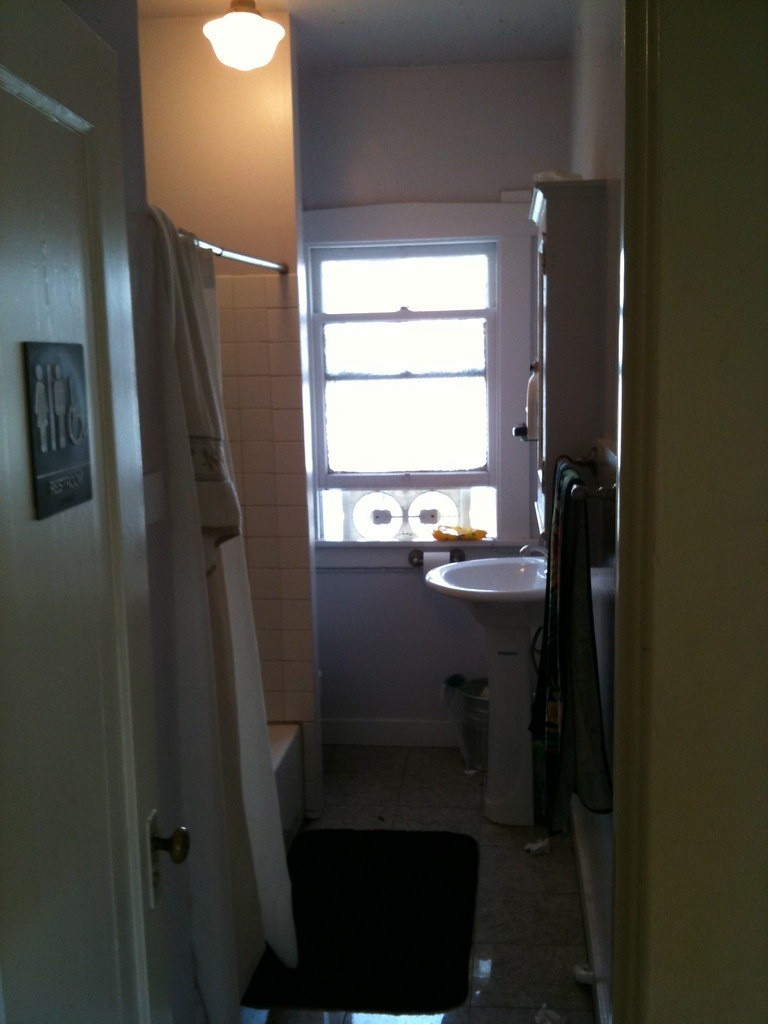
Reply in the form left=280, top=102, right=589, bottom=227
left=423, top=552, right=449, bottom=576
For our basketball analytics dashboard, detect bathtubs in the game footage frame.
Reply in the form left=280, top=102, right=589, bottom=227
left=267, top=722, right=303, bottom=854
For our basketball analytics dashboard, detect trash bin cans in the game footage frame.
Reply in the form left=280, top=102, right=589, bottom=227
left=458, top=678, right=490, bottom=771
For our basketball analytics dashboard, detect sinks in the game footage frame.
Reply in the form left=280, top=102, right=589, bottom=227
left=424, top=557, right=547, bottom=629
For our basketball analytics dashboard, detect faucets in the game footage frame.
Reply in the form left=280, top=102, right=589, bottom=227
left=520, top=545, right=548, bottom=557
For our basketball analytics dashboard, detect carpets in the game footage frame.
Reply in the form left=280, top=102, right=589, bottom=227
left=240, top=828, right=482, bottom=1014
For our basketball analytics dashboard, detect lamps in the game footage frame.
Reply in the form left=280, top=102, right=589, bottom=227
left=203, top=0, right=285, bottom=72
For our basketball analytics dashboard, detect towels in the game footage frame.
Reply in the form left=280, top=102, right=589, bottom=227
left=147, top=204, right=242, bottom=546
left=533, top=456, right=614, bottom=836
